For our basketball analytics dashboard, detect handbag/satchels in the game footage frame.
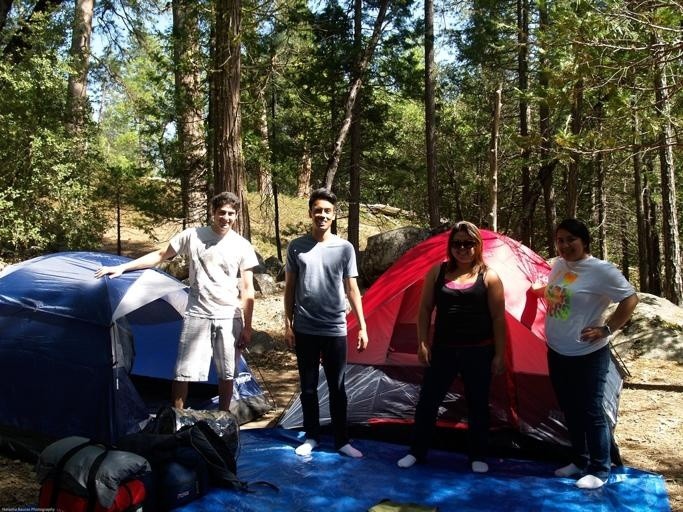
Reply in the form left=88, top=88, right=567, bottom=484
left=39, top=440, right=146, bottom=512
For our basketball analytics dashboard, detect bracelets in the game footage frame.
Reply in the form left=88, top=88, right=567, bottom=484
left=605, top=324, right=613, bottom=335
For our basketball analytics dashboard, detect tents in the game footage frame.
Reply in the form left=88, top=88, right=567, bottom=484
left=0, top=251, right=274, bottom=448
left=278, top=224, right=626, bottom=466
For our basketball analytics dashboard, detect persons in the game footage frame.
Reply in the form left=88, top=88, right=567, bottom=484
left=283, top=186, right=369, bottom=458
left=529, top=217, right=639, bottom=490
left=398, top=220, right=507, bottom=475
left=93, top=191, right=261, bottom=409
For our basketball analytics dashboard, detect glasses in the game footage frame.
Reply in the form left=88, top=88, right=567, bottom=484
left=451, top=240, right=476, bottom=249
